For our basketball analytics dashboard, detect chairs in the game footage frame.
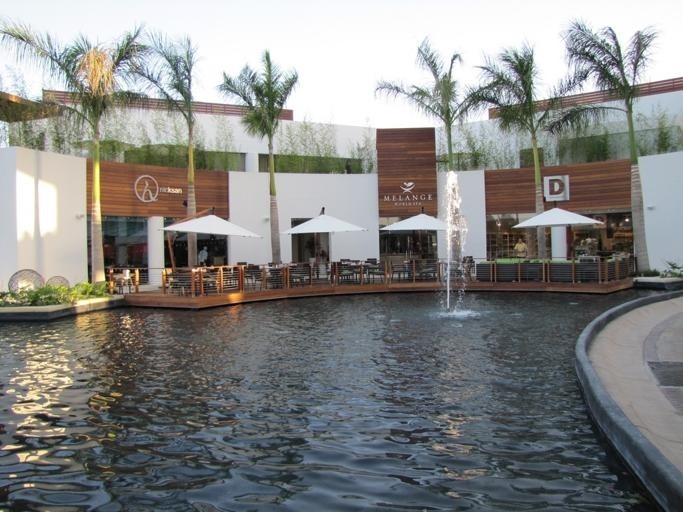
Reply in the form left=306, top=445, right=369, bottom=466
left=336, top=257, right=439, bottom=284
left=164, top=261, right=310, bottom=297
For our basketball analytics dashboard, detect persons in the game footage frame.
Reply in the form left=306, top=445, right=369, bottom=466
left=304, top=241, right=322, bottom=263
left=515, top=238, right=527, bottom=259
left=103, top=242, right=113, bottom=266
left=416, top=242, right=422, bottom=259
left=571, top=234, right=599, bottom=260
left=198, top=246, right=208, bottom=266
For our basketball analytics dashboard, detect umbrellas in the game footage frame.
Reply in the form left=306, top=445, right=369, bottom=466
left=512, top=201, right=604, bottom=229
left=286, top=206, right=367, bottom=233
left=158, top=207, right=263, bottom=238
left=378, top=207, right=459, bottom=231
